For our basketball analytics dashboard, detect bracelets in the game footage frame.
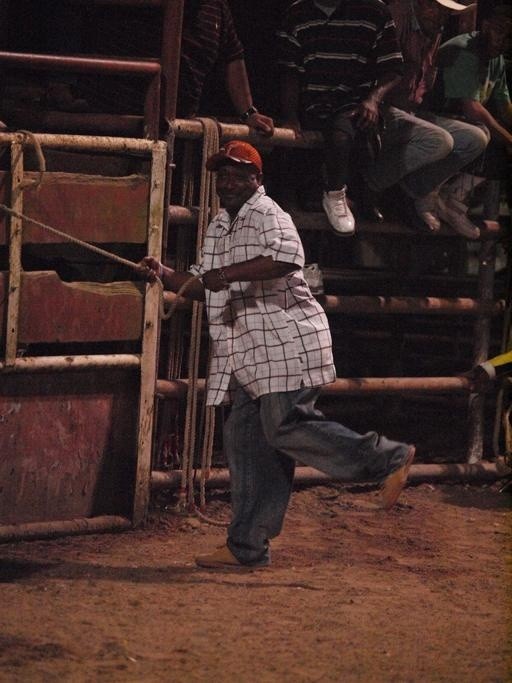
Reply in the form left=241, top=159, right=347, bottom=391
left=215, top=264, right=228, bottom=286
left=238, top=106, right=259, bottom=119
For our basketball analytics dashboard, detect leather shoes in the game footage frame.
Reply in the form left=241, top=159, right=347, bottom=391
left=350, top=180, right=385, bottom=224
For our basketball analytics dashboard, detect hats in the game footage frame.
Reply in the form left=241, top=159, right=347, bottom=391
left=436, top=0, right=478, bottom=17
left=205, top=140, right=263, bottom=173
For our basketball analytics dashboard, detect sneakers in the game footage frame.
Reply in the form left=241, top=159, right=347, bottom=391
left=404, top=190, right=441, bottom=233
left=195, top=543, right=249, bottom=569
left=379, top=444, right=416, bottom=513
left=438, top=198, right=481, bottom=241
left=322, top=184, right=356, bottom=237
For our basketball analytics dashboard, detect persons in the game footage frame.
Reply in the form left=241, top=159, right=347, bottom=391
left=272, top=0, right=409, bottom=236
left=135, top=132, right=414, bottom=571
left=368, top=0, right=485, bottom=238
left=419, top=0, right=511, bottom=234
left=113, top=1, right=280, bottom=139
